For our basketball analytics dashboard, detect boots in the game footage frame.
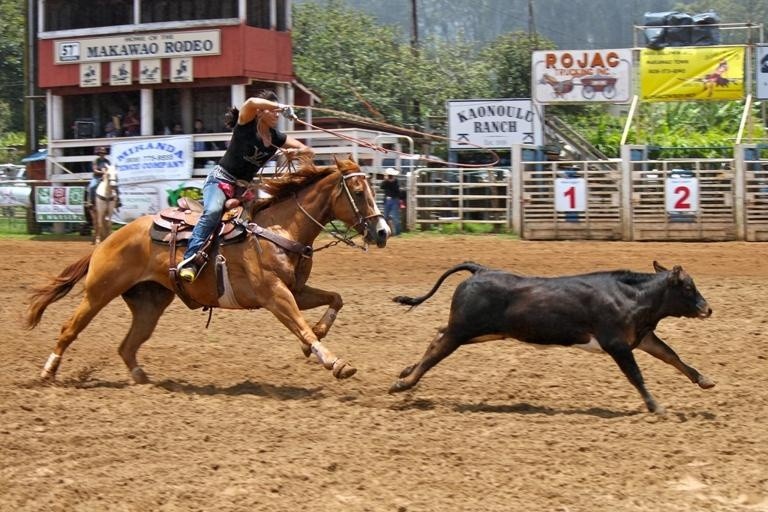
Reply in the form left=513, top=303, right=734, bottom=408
left=115, top=198, right=121, bottom=208
left=84, top=200, right=93, bottom=208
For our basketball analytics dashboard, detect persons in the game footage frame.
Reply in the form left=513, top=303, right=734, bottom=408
left=86, top=147, right=122, bottom=209
left=379, top=168, right=402, bottom=237
left=104, top=103, right=232, bottom=177
left=178, top=89, right=313, bottom=285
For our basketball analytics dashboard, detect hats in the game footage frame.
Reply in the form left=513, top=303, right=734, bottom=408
left=384, top=168, right=399, bottom=176
left=97, top=147, right=107, bottom=153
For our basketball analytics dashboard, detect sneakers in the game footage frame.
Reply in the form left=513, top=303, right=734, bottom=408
left=179, top=264, right=196, bottom=282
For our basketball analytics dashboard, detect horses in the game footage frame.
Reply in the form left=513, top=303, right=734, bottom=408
left=22, top=154, right=392, bottom=384
left=538, top=73, right=577, bottom=99
left=90, top=162, right=119, bottom=244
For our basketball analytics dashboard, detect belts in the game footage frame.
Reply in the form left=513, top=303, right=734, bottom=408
left=216, top=167, right=236, bottom=182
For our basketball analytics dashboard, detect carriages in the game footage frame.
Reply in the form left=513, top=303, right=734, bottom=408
left=538, top=73, right=617, bottom=99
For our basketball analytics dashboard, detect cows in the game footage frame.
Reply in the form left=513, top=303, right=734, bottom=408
left=388, top=261, right=716, bottom=414
